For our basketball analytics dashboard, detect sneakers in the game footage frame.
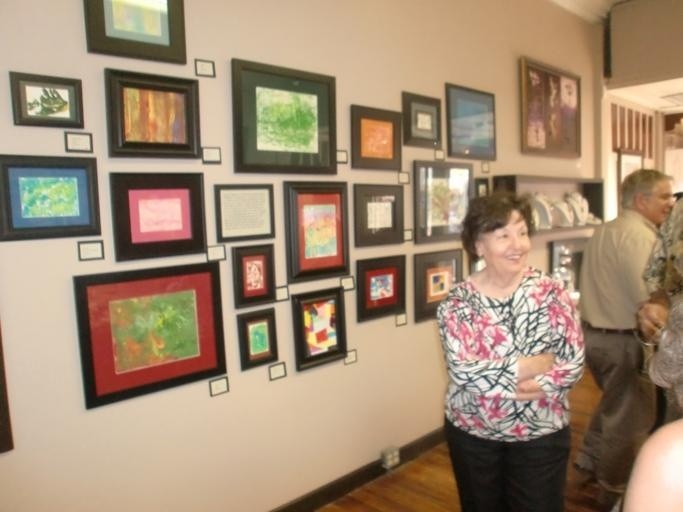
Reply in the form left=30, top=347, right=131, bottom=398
left=574, top=451, right=626, bottom=494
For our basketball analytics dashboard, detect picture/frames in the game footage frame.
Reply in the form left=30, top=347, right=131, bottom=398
left=107, top=169, right=210, bottom=264
left=212, top=182, right=278, bottom=245
left=229, top=243, right=279, bottom=310
left=354, top=252, right=409, bottom=325
left=413, top=248, right=466, bottom=327
left=235, top=307, right=282, bottom=373
left=442, top=79, right=498, bottom=165
left=411, top=157, right=477, bottom=247
left=517, top=54, right=583, bottom=162
left=228, top=56, right=340, bottom=177
left=348, top=101, right=405, bottom=175
left=81, top=1, right=189, bottom=68
left=8, top=68, right=86, bottom=132
left=101, top=65, right=205, bottom=161
left=71, top=259, right=229, bottom=411
left=400, top=88, right=444, bottom=152
left=1, top=152, right=105, bottom=241
left=282, top=178, right=353, bottom=285
left=288, top=284, right=349, bottom=373
left=351, top=181, right=407, bottom=249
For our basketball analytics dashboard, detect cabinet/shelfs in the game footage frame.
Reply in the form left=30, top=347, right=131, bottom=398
left=491, top=175, right=605, bottom=295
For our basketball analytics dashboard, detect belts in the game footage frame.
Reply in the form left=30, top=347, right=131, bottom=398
left=581, top=320, right=641, bottom=335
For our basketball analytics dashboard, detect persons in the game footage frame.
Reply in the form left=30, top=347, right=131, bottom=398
left=435, top=192, right=586, bottom=512
left=635, top=194, right=683, bottom=421
left=622, top=286, right=682, bottom=512
left=575, top=167, right=673, bottom=506
